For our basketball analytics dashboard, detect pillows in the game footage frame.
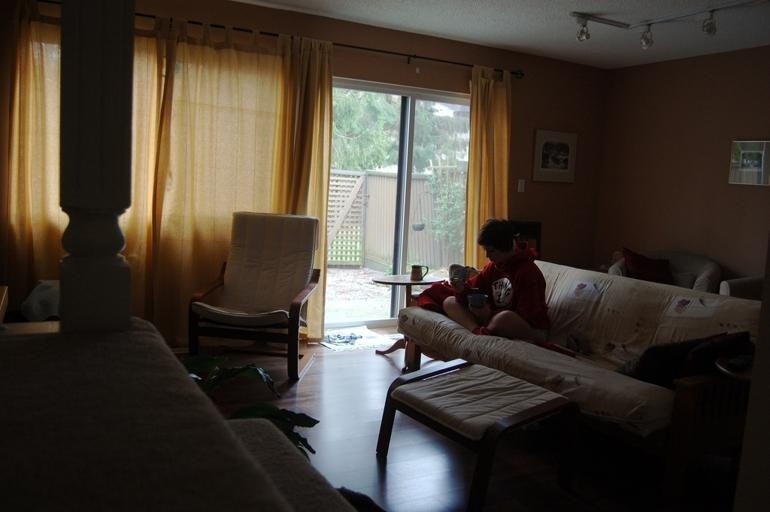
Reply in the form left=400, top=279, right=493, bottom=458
left=615, top=328, right=757, bottom=393
left=622, top=248, right=698, bottom=289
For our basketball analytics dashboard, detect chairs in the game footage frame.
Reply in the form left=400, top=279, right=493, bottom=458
left=608, top=249, right=722, bottom=293
left=186, top=210, right=322, bottom=380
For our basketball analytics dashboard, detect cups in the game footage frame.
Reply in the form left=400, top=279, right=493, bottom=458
left=410, top=265, right=429, bottom=281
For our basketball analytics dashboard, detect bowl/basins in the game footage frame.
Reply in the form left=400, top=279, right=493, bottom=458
left=467, top=294, right=489, bottom=308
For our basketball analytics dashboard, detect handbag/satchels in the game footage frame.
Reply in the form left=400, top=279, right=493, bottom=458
left=417, top=281, right=457, bottom=313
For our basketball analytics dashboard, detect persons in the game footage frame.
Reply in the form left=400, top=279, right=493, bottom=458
left=441, top=218, right=550, bottom=341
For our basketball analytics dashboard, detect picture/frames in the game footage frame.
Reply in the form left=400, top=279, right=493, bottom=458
left=532, top=128, right=577, bottom=184
left=727, top=139, right=770, bottom=187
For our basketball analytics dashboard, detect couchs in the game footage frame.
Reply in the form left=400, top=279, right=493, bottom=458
left=398, top=261, right=763, bottom=446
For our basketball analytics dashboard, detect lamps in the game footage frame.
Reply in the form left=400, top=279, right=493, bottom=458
left=570, top=11, right=718, bottom=53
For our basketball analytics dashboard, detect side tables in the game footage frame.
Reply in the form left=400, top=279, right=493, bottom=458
left=374, top=274, right=446, bottom=355
